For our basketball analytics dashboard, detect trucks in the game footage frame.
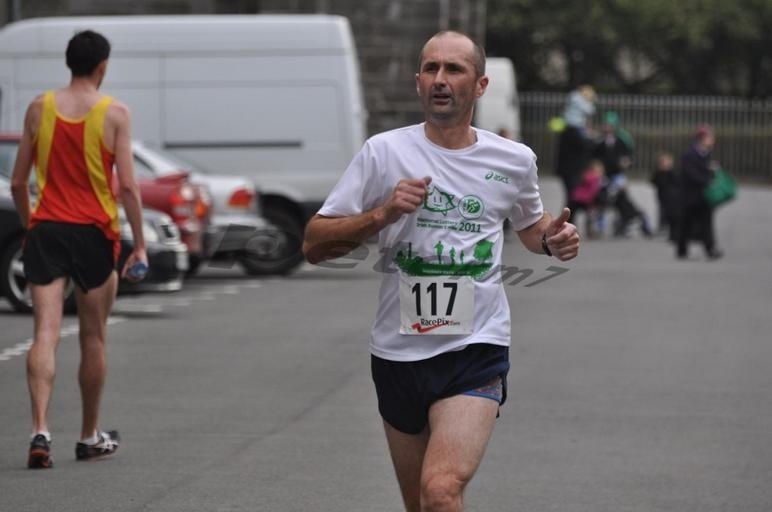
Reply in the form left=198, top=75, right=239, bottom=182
left=0, top=11, right=370, bottom=281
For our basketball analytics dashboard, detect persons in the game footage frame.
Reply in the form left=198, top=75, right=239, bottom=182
left=651, top=153, right=679, bottom=232
left=605, top=153, right=651, bottom=237
left=595, top=110, right=636, bottom=176
left=557, top=83, right=598, bottom=168
left=668, top=127, right=723, bottom=258
left=11, top=29, right=152, bottom=469
left=304, top=30, right=581, bottom=512
left=565, top=158, right=606, bottom=237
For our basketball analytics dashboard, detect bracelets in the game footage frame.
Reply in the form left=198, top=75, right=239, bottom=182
left=541, top=233, right=553, bottom=256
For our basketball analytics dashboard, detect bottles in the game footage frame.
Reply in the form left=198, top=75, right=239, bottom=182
left=125, top=260, right=148, bottom=280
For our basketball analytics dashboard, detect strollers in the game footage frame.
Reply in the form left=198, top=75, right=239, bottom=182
left=609, top=185, right=653, bottom=239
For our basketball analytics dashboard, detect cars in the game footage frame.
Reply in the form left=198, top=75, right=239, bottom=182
left=1, top=130, right=260, bottom=264
left=0, top=171, right=192, bottom=317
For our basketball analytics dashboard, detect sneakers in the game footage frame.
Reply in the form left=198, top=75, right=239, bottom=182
left=75, top=430, right=120, bottom=460
left=27, top=434, right=55, bottom=468
